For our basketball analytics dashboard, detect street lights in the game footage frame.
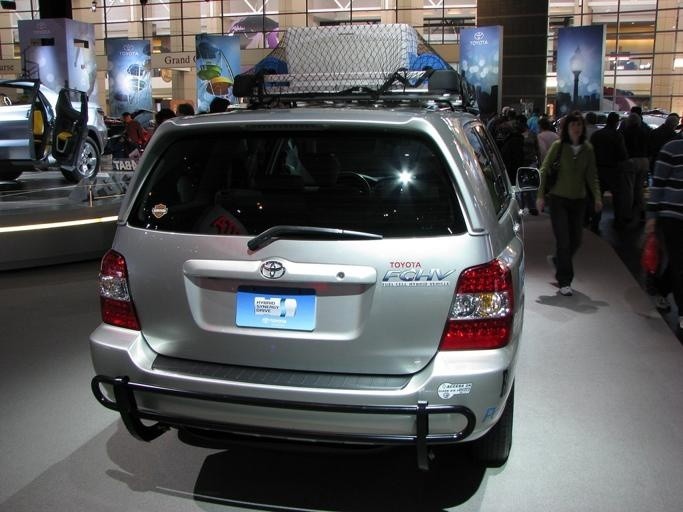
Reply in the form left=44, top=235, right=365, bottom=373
left=569, top=45, right=585, bottom=108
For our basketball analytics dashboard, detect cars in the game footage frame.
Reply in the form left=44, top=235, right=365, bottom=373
left=550, top=110, right=681, bottom=136
left=0, top=77, right=109, bottom=184
left=101, top=110, right=153, bottom=155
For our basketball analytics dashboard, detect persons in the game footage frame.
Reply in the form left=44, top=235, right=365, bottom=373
left=121, top=96, right=230, bottom=149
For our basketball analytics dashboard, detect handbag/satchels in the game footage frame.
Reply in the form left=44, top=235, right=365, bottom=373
left=639, top=226, right=670, bottom=280
left=544, top=138, right=563, bottom=194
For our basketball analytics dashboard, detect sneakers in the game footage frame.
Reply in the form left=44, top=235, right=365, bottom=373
left=546, top=255, right=557, bottom=274
left=656, top=297, right=673, bottom=313
left=559, top=285, right=573, bottom=296
left=676, top=315, right=683, bottom=333
left=528, top=207, right=540, bottom=217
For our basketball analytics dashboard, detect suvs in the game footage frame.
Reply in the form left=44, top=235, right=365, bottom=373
left=84, top=70, right=542, bottom=477
